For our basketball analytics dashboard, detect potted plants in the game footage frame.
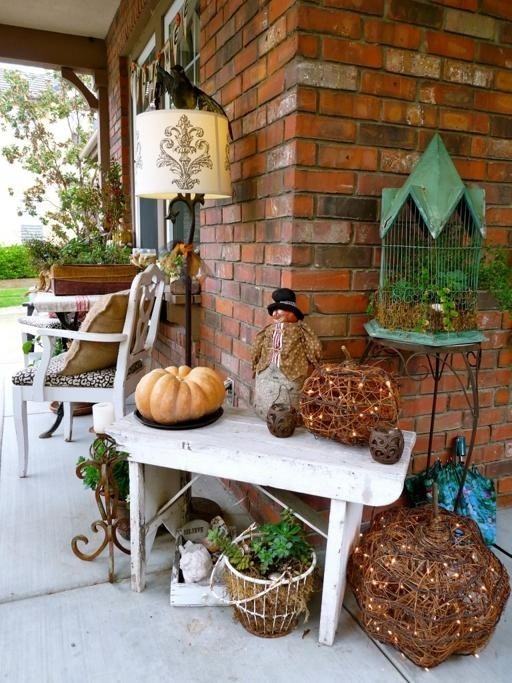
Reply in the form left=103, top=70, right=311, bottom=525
left=205, top=507, right=316, bottom=638
left=78, top=435, right=130, bottom=542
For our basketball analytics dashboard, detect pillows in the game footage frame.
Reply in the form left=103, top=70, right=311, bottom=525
left=56, top=290, right=150, bottom=376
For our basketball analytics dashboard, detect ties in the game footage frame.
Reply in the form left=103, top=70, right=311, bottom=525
left=272, top=323, right=282, bottom=368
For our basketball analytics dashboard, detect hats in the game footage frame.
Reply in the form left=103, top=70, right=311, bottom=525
left=267, top=288, right=306, bottom=321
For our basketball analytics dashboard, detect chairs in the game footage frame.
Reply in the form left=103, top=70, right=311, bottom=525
left=10, top=263, right=166, bottom=479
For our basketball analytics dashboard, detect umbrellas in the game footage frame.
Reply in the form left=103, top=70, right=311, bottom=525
left=402, top=430, right=497, bottom=551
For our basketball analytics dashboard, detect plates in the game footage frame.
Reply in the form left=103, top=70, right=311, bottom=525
left=130, top=406, right=224, bottom=433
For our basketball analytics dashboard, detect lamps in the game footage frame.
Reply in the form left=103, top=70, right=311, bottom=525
left=133, top=108, right=233, bottom=369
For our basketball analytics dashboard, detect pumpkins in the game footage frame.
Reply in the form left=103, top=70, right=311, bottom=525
left=135, top=365, right=226, bottom=425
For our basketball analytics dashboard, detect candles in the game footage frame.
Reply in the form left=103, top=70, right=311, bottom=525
left=92, top=402, right=115, bottom=434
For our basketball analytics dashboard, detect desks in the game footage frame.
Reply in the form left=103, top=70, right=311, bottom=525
left=106, top=404, right=417, bottom=647
